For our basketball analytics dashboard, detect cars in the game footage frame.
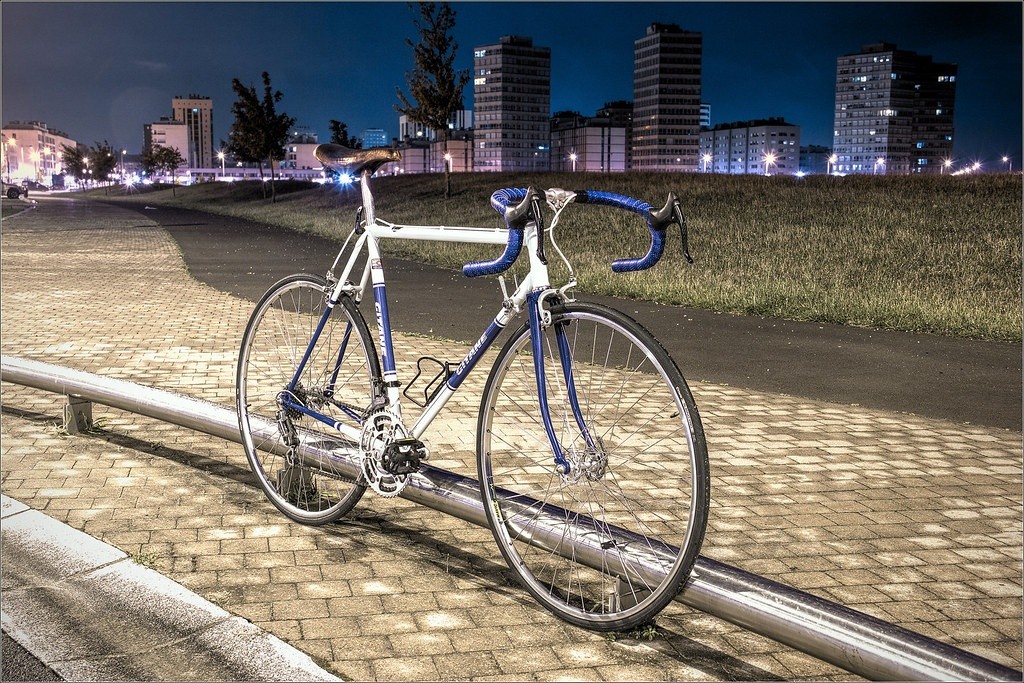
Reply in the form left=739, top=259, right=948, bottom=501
left=0, top=179, right=25, bottom=199
left=28, top=182, right=48, bottom=192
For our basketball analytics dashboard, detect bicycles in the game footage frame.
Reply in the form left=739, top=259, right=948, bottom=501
left=235, top=142, right=712, bottom=633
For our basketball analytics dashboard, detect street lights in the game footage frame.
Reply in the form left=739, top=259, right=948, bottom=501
left=940, top=159, right=952, bottom=174
left=1003, top=157, right=1012, bottom=171
left=874, top=158, right=884, bottom=175
left=570, top=152, right=576, bottom=171
left=702, top=154, right=710, bottom=173
left=120, top=149, right=127, bottom=184
left=827, top=155, right=836, bottom=173
left=217, top=151, right=226, bottom=180
left=765, top=153, right=775, bottom=174
left=444, top=153, right=453, bottom=172
